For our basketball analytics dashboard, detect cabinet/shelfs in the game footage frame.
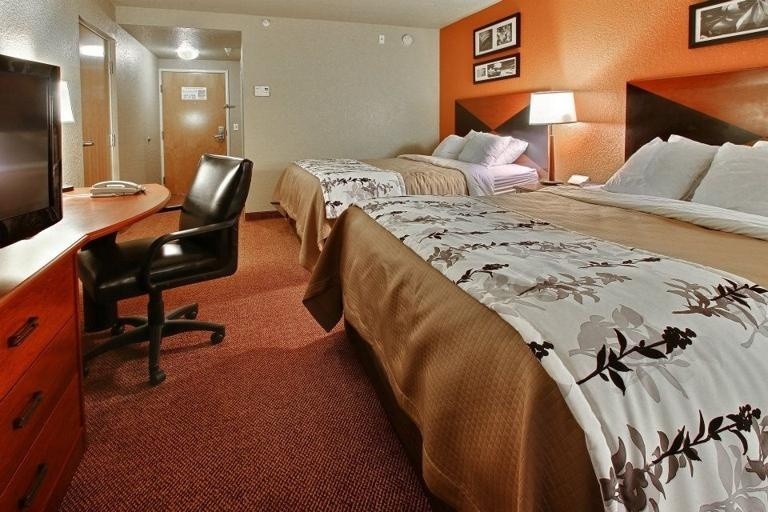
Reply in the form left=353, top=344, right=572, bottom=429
left=1, top=250, right=89, bottom=512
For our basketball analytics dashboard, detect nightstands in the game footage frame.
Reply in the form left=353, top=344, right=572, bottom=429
left=513, top=181, right=549, bottom=193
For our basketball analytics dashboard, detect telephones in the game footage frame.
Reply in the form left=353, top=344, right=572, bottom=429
left=91, top=181, right=148, bottom=198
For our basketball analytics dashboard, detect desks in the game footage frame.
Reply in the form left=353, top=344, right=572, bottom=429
left=62, top=182, right=170, bottom=240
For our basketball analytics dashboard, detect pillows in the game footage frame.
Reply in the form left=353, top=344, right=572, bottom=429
left=753, top=139, right=768, bottom=154
left=667, top=130, right=721, bottom=199
left=600, top=136, right=720, bottom=199
left=463, top=128, right=480, bottom=140
left=432, top=134, right=467, bottom=159
left=457, top=132, right=513, bottom=166
left=493, top=137, right=529, bottom=167
left=691, top=141, right=768, bottom=217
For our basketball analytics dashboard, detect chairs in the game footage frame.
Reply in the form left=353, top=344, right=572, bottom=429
left=74, top=154, right=254, bottom=384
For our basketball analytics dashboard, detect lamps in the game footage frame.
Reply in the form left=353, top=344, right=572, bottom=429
left=529, top=90, right=576, bottom=185
left=59, top=81, right=75, bottom=123
left=178, top=38, right=201, bottom=60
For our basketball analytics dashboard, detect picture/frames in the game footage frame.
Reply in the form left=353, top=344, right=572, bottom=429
left=688, top=0, right=768, bottom=49
left=473, top=12, right=520, bottom=59
left=472, top=52, right=520, bottom=84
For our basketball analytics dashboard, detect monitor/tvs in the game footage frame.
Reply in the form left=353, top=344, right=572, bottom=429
left=0, top=53, right=63, bottom=250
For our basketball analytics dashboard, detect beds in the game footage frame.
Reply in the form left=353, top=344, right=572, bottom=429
left=270, top=91, right=551, bottom=275
left=303, top=66, right=768, bottom=511
